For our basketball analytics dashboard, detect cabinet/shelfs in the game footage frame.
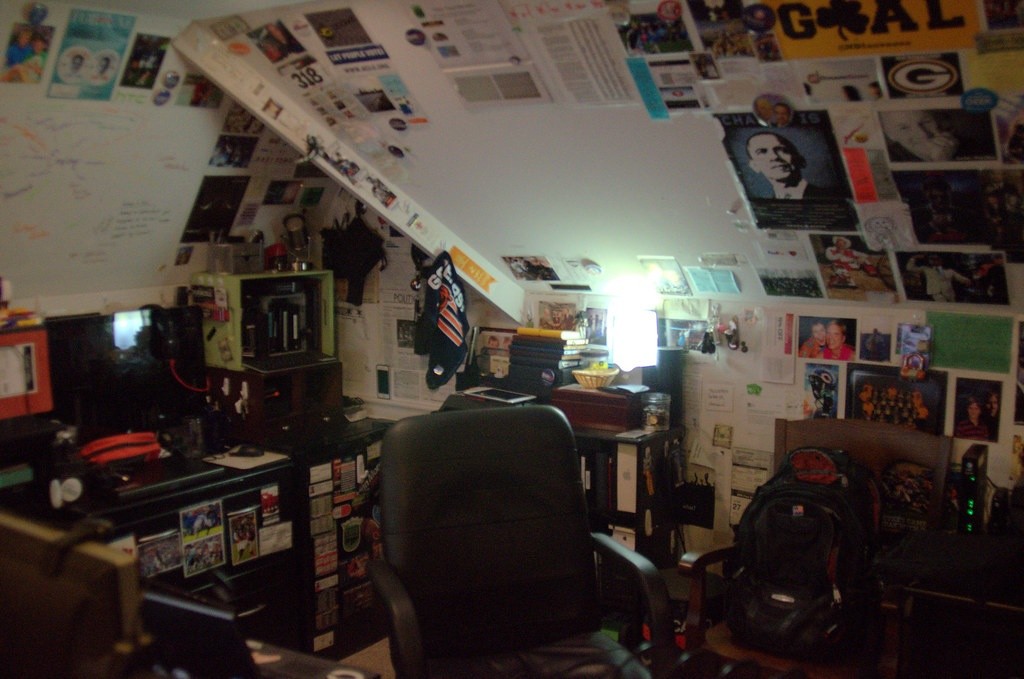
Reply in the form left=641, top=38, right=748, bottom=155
left=439, top=394, right=682, bottom=587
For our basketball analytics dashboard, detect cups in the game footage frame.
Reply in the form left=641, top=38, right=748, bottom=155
left=641, top=393, right=670, bottom=431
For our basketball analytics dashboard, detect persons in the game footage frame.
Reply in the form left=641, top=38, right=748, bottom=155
left=879, top=109, right=959, bottom=161
left=185, top=505, right=257, bottom=576
left=90, top=57, right=110, bottom=79
left=767, top=102, right=794, bottom=128
left=502, top=336, right=512, bottom=349
left=799, top=319, right=855, bottom=361
left=65, top=54, right=85, bottom=77
left=5, top=29, right=45, bottom=68
left=824, top=237, right=871, bottom=271
left=907, top=253, right=971, bottom=303
left=746, top=131, right=825, bottom=199
left=956, top=392, right=999, bottom=441
left=487, top=336, right=499, bottom=348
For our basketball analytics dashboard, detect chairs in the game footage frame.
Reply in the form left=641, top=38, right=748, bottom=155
left=364, top=405, right=678, bottom=679
left=707, top=418, right=952, bottom=679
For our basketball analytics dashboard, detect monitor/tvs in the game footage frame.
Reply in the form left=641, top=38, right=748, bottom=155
left=111, top=308, right=154, bottom=352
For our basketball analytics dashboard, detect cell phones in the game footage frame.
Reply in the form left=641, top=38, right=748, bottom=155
left=376, top=364, right=391, bottom=400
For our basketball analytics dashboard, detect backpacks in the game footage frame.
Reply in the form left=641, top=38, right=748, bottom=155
left=724, top=446, right=879, bottom=662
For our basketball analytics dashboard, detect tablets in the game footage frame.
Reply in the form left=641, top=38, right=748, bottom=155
left=463, top=385, right=537, bottom=404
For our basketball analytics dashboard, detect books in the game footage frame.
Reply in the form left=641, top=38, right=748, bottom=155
left=509, top=327, right=589, bottom=368
